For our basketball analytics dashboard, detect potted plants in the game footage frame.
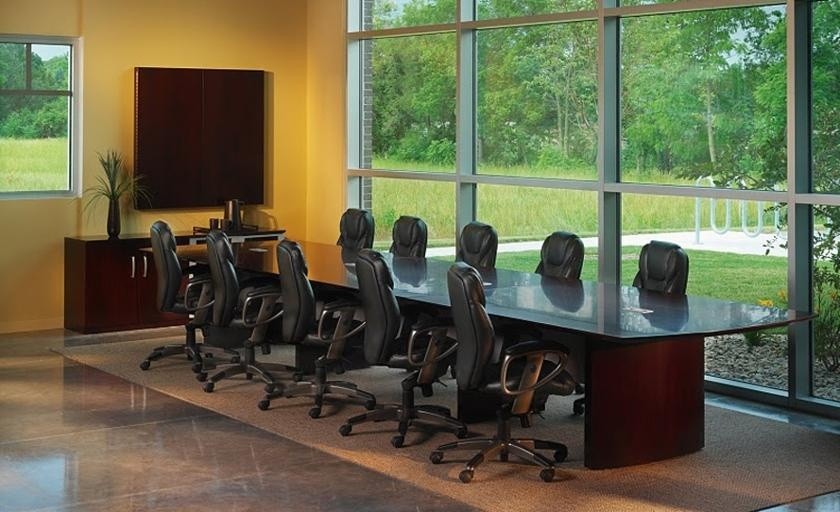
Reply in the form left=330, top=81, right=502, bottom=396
left=69, top=150, right=153, bottom=237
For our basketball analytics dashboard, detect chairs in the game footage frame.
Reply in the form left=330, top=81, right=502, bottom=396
left=137, top=221, right=236, bottom=372
left=632, top=241, right=689, bottom=294
left=256, top=241, right=377, bottom=418
left=339, top=249, right=470, bottom=448
left=391, top=216, right=424, bottom=254
left=456, top=221, right=498, bottom=269
left=191, top=231, right=304, bottom=392
left=534, top=231, right=584, bottom=278
left=429, top=262, right=572, bottom=482
left=337, top=208, right=374, bottom=250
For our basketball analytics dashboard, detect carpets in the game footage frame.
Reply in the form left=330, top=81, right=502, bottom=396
left=48, top=325, right=840, bottom=511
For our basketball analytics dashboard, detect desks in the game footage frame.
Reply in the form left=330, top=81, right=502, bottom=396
left=137, top=241, right=818, bottom=471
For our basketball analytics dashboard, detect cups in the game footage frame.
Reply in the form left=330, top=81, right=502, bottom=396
left=221, top=219, right=232, bottom=231
left=210, top=218, right=218, bottom=229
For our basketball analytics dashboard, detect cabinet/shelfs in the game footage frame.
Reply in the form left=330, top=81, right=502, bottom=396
left=63, top=235, right=192, bottom=334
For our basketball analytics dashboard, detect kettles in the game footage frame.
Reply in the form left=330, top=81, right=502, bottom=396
left=224, top=199, right=245, bottom=231
